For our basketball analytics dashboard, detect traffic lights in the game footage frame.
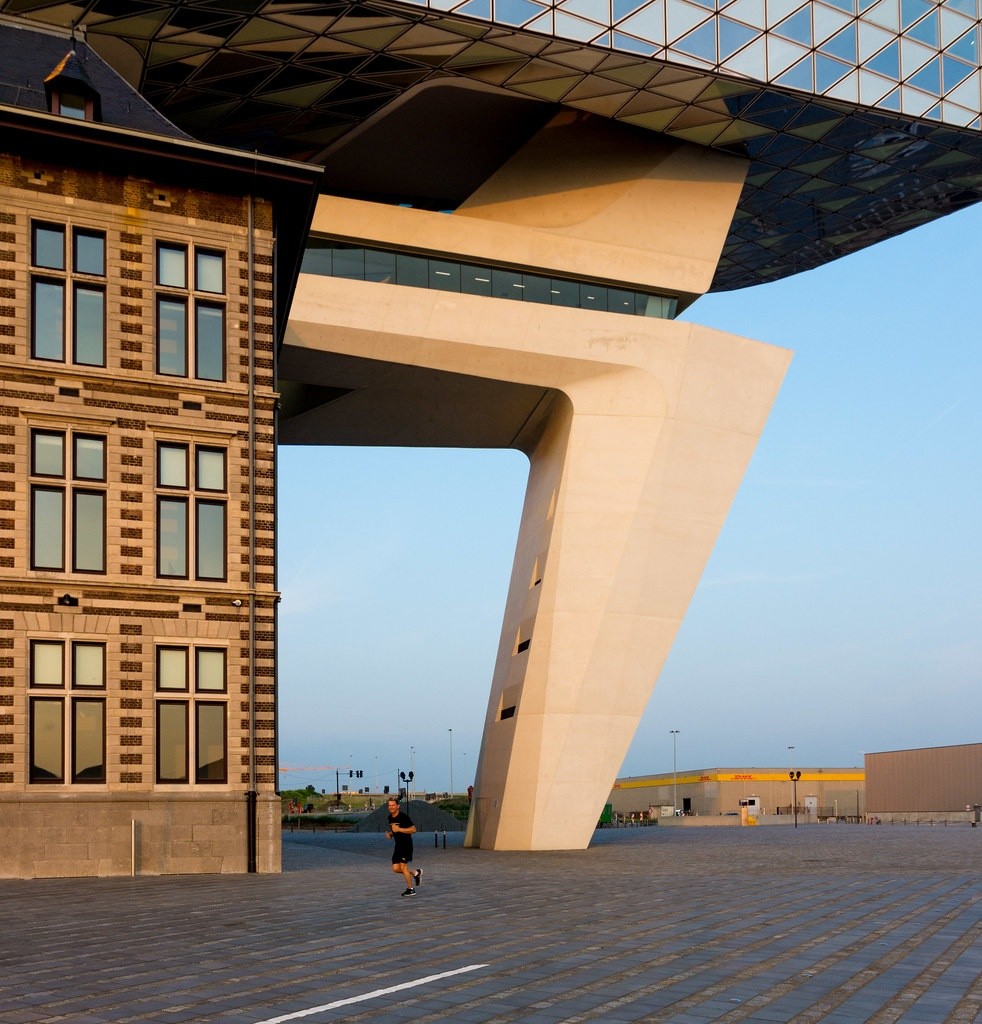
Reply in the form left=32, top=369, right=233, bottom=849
left=360, top=770, right=363, bottom=778
left=356, top=770, right=359, bottom=778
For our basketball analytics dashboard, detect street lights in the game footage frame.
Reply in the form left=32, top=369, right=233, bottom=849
left=449, top=728, right=452, bottom=799
left=670, top=731, right=680, bottom=816
left=790, top=771, right=801, bottom=828
left=400, top=771, right=414, bottom=818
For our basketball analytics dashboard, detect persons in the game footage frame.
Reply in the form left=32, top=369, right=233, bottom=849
left=386, top=797, right=423, bottom=897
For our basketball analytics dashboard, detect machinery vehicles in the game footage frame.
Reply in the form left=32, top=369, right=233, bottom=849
left=287, top=797, right=313, bottom=813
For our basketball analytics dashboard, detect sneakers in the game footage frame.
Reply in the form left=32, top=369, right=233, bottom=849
left=413, top=868, right=424, bottom=887
left=400, top=888, right=416, bottom=897
